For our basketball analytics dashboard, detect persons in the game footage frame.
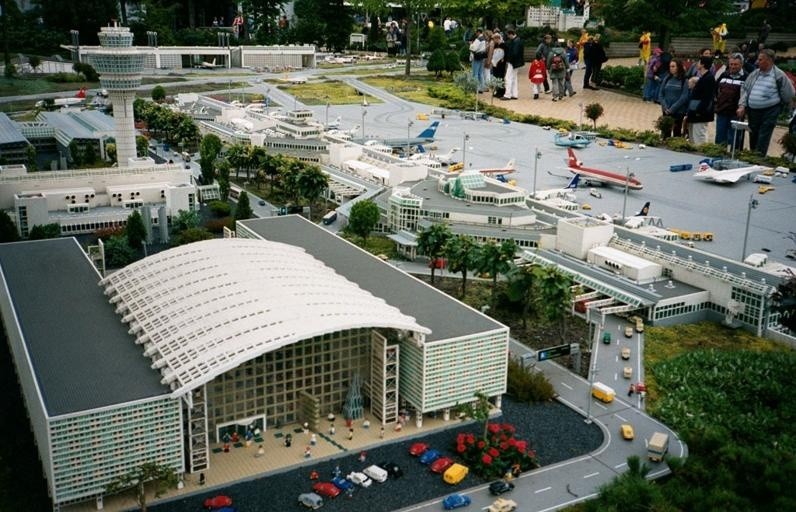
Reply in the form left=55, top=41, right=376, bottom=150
left=503, top=462, right=521, bottom=483
left=358, top=14, right=610, bottom=102
left=201, top=408, right=407, bottom=498
left=628, top=383, right=647, bottom=401
left=211, top=14, right=290, bottom=37
left=638, top=18, right=796, bottom=163
